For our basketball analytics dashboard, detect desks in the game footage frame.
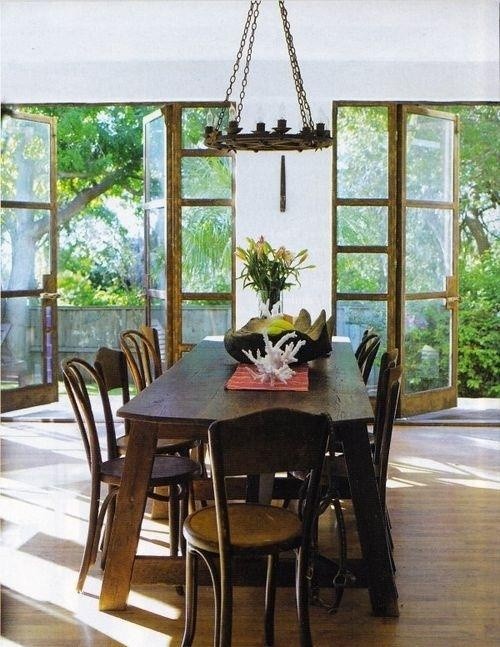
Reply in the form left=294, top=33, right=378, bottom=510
left=97, top=333, right=402, bottom=616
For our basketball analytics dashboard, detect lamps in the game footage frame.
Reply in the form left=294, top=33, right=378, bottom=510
left=204, top=1, right=336, bottom=154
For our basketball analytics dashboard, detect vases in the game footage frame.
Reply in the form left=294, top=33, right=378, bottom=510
left=254, top=284, right=285, bottom=318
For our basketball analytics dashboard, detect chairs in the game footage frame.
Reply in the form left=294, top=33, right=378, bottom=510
left=60, top=325, right=204, bottom=595
left=181, top=403, right=333, bottom=647
left=280, top=329, right=402, bottom=614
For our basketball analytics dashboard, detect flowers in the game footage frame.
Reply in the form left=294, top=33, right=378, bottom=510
left=231, top=231, right=317, bottom=314
